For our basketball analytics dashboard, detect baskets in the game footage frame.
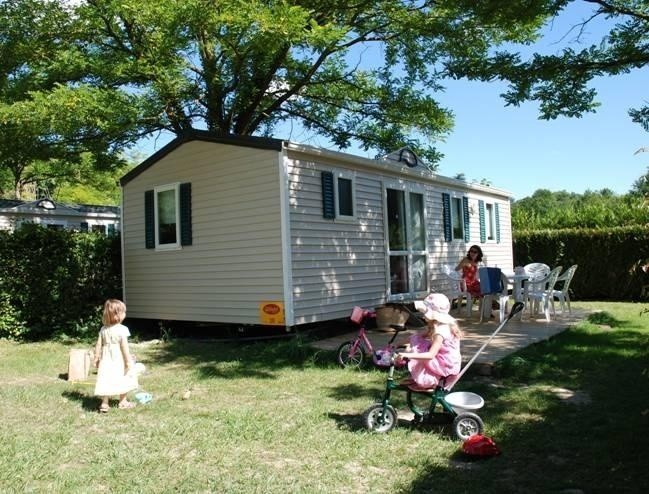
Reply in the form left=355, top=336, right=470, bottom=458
left=375, top=301, right=410, bottom=332
left=350, top=305, right=364, bottom=324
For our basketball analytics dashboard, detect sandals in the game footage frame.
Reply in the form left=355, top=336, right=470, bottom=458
left=100, top=402, right=109, bottom=412
left=118, top=401, right=136, bottom=408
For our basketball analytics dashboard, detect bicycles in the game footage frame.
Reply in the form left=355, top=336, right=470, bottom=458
left=338, top=307, right=411, bottom=370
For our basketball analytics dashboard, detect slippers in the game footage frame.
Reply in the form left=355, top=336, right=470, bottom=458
left=400, top=378, right=434, bottom=392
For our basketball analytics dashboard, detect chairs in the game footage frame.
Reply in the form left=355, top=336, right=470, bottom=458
left=441, top=261, right=579, bottom=324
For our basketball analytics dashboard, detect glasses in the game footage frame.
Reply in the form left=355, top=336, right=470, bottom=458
left=470, top=250, right=478, bottom=254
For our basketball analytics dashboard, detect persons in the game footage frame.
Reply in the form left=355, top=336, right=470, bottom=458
left=455, top=245, right=500, bottom=317
left=93, top=298, right=138, bottom=412
left=392, top=292, right=463, bottom=392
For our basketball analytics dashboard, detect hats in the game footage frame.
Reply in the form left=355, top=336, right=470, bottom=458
left=414, top=293, right=455, bottom=324
